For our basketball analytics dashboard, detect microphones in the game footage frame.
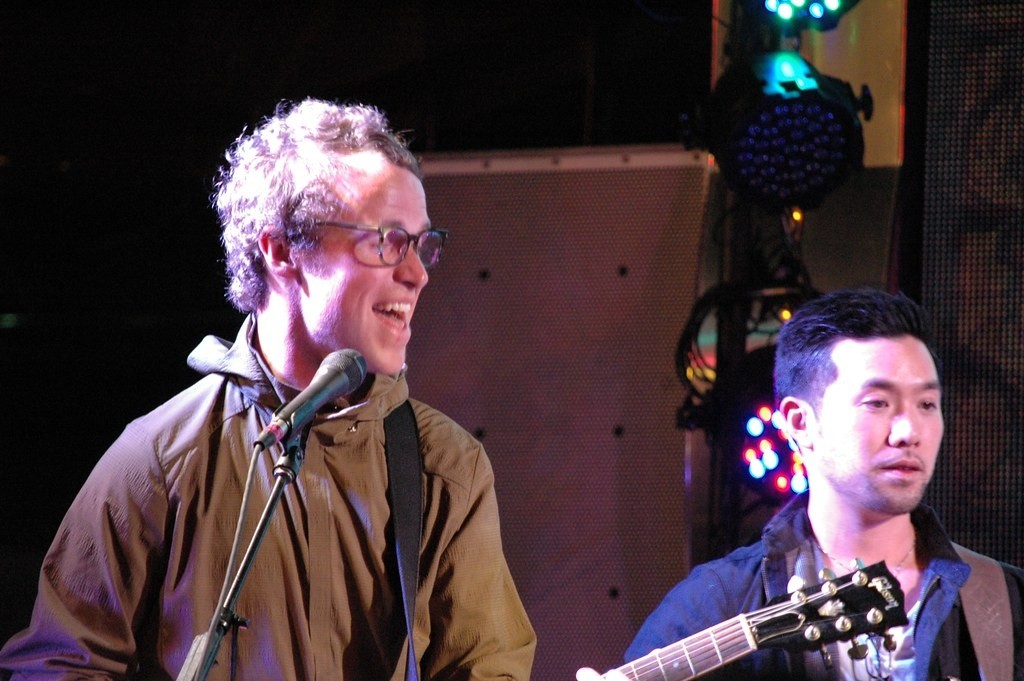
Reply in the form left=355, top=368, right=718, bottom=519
left=253, top=348, right=367, bottom=451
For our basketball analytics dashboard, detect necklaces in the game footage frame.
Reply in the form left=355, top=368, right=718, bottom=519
left=811, top=531, right=917, bottom=577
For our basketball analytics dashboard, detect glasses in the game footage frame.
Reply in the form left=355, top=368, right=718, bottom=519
left=315, top=221, right=448, bottom=268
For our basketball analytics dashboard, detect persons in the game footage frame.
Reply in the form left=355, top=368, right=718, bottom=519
left=0, top=96, right=628, bottom=681
left=618, top=289, right=1023, bottom=681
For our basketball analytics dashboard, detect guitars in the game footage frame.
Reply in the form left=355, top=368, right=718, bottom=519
left=598, top=554, right=908, bottom=681
